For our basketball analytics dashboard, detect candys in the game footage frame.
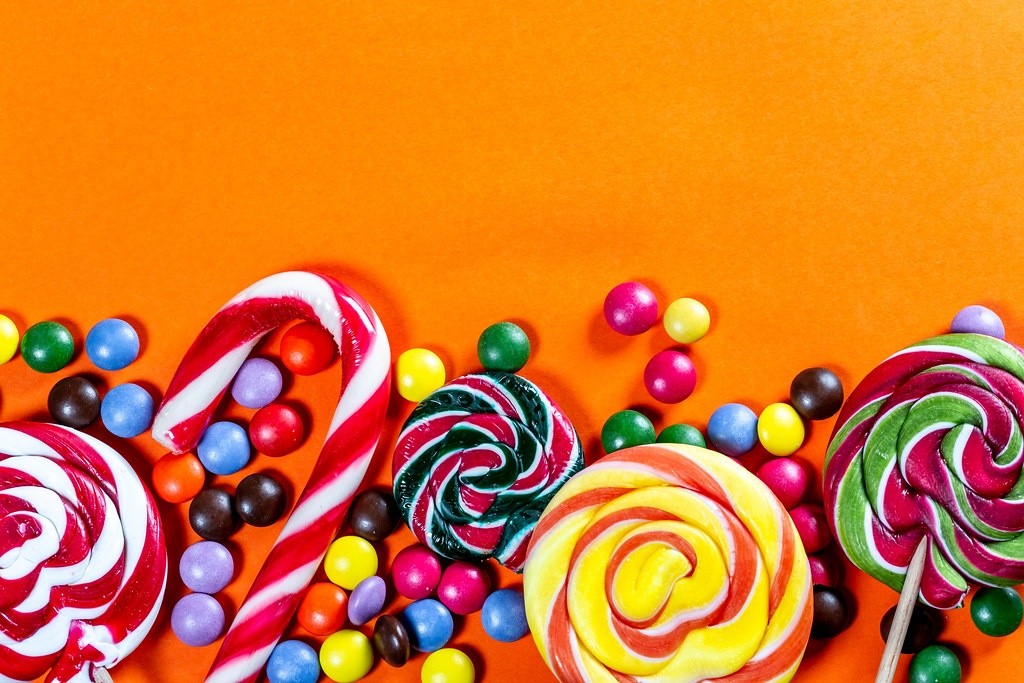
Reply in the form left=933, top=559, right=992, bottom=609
left=0, top=270, right=1024, bottom=683
